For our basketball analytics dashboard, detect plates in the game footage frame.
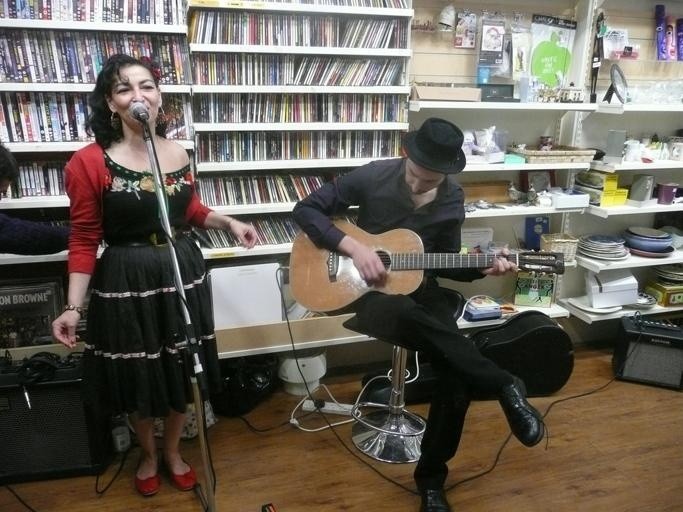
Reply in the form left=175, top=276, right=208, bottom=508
left=577, top=234, right=632, bottom=262
left=620, top=291, right=657, bottom=310
left=620, top=225, right=676, bottom=258
left=651, top=266, right=683, bottom=283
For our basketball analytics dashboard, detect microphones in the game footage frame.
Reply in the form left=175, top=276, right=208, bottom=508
left=126, top=100, right=153, bottom=122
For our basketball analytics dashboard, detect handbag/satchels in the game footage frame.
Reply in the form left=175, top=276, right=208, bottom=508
left=208, top=354, right=281, bottom=417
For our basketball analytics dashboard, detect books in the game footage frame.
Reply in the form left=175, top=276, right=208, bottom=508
left=0, top=0, right=410, bottom=255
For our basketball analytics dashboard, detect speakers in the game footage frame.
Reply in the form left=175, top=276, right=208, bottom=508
left=610, top=315, right=683, bottom=392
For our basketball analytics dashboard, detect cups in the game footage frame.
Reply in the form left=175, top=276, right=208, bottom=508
left=656, top=178, right=679, bottom=205
left=562, top=82, right=585, bottom=103
left=624, top=81, right=682, bottom=103
left=630, top=174, right=654, bottom=200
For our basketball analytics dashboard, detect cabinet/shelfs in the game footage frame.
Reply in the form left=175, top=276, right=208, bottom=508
left=0, top=0, right=192, bottom=267
left=187, top=0, right=414, bottom=260
left=555, top=103, right=683, bottom=324
left=405, top=96, right=598, bottom=327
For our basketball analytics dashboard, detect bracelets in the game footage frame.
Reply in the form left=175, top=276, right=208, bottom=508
left=63, top=304, right=84, bottom=319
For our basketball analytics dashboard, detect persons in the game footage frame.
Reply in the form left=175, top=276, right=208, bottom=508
left=49, top=53, right=257, bottom=498
left=292, top=118, right=546, bottom=512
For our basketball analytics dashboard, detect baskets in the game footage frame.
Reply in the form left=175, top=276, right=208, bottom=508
left=507, top=144, right=596, bottom=165
left=539, top=232, right=579, bottom=261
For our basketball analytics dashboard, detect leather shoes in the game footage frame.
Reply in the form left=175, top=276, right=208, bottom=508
left=497, top=376, right=544, bottom=447
left=419, top=474, right=450, bottom=512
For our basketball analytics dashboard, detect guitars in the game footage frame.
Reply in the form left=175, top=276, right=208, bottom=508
left=290, top=216, right=564, bottom=317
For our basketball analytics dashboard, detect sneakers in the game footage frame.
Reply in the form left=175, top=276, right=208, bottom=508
left=168, top=457, right=197, bottom=491
left=136, top=456, right=160, bottom=496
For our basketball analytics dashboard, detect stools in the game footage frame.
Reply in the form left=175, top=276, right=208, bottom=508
left=343, top=284, right=468, bottom=465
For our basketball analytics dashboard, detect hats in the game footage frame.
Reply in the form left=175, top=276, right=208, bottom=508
left=401, top=117, right=465, bottom=174
